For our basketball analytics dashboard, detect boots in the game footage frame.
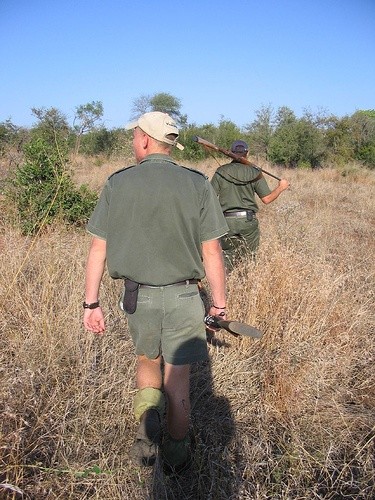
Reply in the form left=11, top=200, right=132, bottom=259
left=131, top=388, right=163, bottom=466
left=162, top=436, right=196, bottom=480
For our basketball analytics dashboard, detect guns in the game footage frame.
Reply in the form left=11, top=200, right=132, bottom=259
left=194, top=137, right=281, bottom=182
left=204, top=315, right=265, bottom=339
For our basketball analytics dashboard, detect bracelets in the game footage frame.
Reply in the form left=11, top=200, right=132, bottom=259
left=213, top=304, right=226, bottom=309
left=83, top=301, right=99, bottom=309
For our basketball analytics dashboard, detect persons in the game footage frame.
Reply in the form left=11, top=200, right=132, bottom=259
left=83, top=111, right=229, bottom=481
left=210, top=141, right=289, bottom=276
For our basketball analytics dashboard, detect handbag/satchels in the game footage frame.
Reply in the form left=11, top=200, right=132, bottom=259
left=123, top=279, right=139, bottom=315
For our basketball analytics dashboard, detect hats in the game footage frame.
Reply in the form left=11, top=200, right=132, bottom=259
left=232, top=141, right=249, bottom=153
left=125, top=111, right=184, bottom=151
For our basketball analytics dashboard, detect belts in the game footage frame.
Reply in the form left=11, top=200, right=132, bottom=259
left=137, top=280, right=198, bottom=287
left=224, top=211, right=255, bottom=216
